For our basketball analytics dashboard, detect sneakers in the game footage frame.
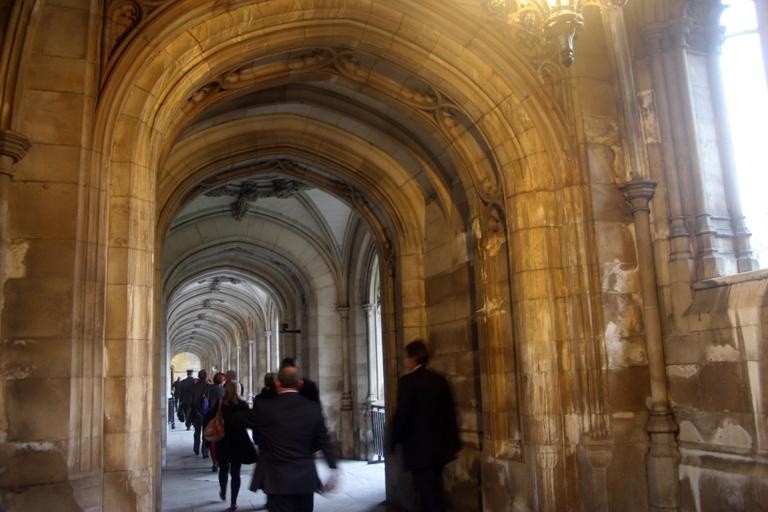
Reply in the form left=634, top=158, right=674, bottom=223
left=211, top=464, right=219, bottom=473
left=220, top=491, right=239, bottom=510
left=193, top=449, right=209, bottom=458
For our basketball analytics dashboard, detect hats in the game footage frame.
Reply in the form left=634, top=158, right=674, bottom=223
left=187, top=369, right=196, bottom=375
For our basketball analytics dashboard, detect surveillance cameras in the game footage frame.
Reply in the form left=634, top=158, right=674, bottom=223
left=283, top=323, right=288, bottom=328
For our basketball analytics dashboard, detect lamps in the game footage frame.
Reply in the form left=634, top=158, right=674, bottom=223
left=487, top=0, right=629, bottom=69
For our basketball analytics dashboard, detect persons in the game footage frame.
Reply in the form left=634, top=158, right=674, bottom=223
left=382, top=337, right=465, bottom=512
left=170, top=353, right=340, bottom=512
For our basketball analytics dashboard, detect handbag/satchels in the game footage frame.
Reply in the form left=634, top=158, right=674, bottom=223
left=203, top=397, right=226, bottom=443
